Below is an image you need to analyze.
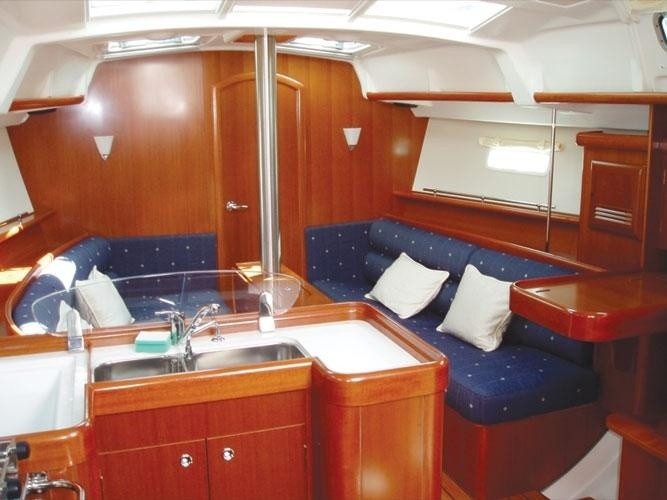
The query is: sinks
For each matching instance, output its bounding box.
[91,326,310,381]
[0,350,89,438]
[292,319,421,374]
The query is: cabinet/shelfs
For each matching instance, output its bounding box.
[510,103,667,500]
[92,390,317,500]
[587,159,645,241]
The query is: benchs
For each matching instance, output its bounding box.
[3,232,234,336]
[302,214,609,500]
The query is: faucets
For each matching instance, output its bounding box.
[65,307,86,353]
[257,290,276,333]
[155,301,221,356]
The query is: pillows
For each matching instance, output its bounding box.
[364,252,450,320]
[55,299,92,332]
[74,265,136,328]
[436,264,515,352]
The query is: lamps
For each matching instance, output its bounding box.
[343,127,362,151]
[94,135,114,161]
